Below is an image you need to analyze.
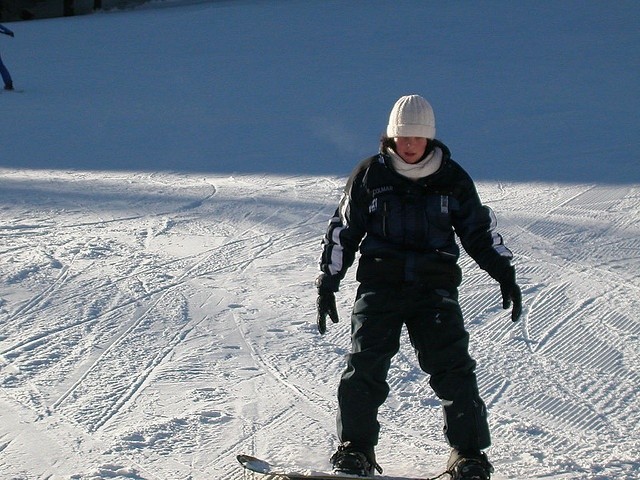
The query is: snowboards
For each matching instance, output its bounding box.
[237,455,437,479]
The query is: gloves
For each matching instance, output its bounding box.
[497,266,522,322]
[315,280,339,334]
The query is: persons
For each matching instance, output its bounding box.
[314,94,522,480]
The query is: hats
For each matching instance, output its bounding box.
[386,94,436,140]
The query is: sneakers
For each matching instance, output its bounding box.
[330,440,383,478]
[446,450,495,480]
